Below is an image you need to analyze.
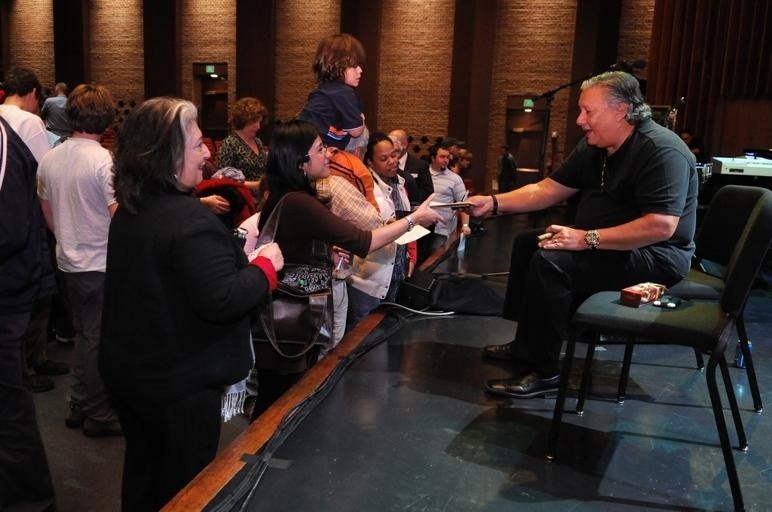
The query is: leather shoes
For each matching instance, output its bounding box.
[483,341,515,361]
[82,416,112,436]
[35,364,49,374]
[30,373,55,393]
[64,409,83,428]
[485,369,561,399]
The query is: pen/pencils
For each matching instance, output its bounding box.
[336,257,344,271]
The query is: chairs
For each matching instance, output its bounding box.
[610,184,772,414]
[547,131,568,177]
[543,191,772,512]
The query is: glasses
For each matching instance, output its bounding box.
[305,145,328,157]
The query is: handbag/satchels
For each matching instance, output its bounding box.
[251,191,334,359]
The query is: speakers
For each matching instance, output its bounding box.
[191,62,228,140]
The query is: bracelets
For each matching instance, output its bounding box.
[492,193,498,216]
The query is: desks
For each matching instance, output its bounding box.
[695,174,772,298]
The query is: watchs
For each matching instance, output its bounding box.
[585,230,602,249]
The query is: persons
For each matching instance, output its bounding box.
[496,144,519,193]
[100,97,473,511]
[1,64,119,511]
[464,71,701,397]
[296,33,366,149]
[677,128,704,159]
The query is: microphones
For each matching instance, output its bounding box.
[673,97,686,109]
[611,59,647,72]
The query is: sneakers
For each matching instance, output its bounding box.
[55,333,79,341]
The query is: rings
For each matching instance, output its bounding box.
[553,238,558,244]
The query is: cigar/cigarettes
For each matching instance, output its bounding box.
[535,232,553,240]
